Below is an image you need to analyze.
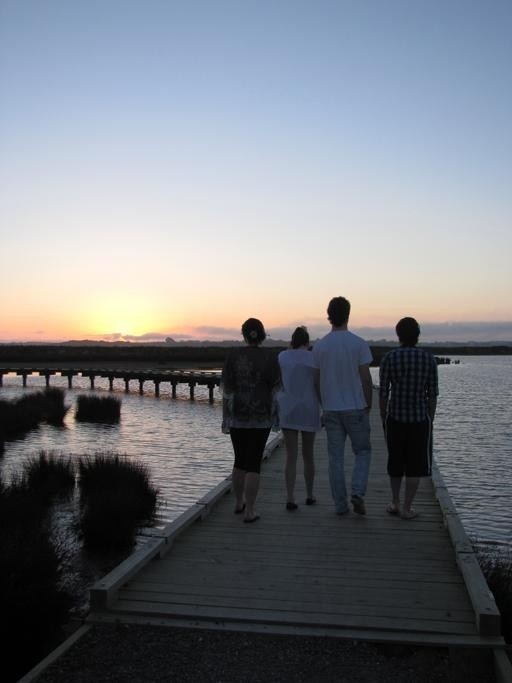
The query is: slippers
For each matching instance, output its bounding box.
[233,502,246,514]
[242,513,261,524]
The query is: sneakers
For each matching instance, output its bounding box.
[400,508,418,520]
[336,507,351,516]
[351,496,366,515]
[386,503,400,514]
[305,495,317,505]
[286,502,298,510]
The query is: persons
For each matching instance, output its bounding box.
[379,315,440,521]
[221,296,372,524]
